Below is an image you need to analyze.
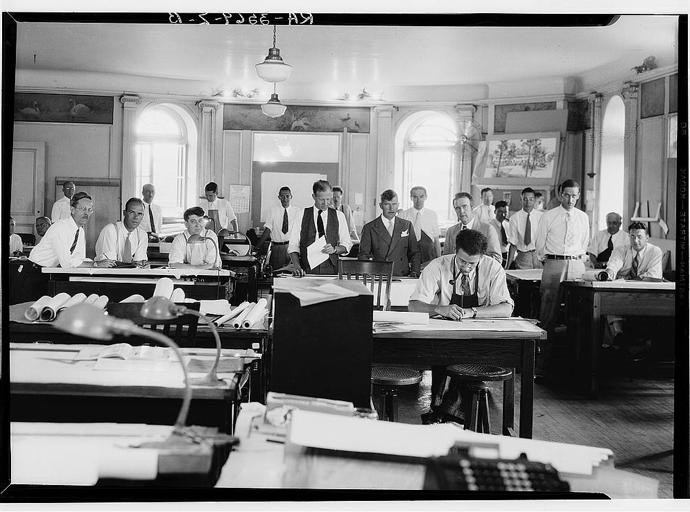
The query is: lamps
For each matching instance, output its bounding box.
[139,295,223,387]
[218,228,253,258]
[203,216,216,234]
[260,80,288,119]
[52,299,209,454]
[186,234,219,270]
[254,26,294,82]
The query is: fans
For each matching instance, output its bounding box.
[442,116,482,192]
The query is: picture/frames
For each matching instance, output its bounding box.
[481,135,560,184]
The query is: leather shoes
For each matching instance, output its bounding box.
[430,395,442,411]
[534,375,545,383]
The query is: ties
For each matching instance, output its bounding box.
[388,221,394,237]
[149,205,156,233]
[70,229,80,255]
[461,275,471,296]
[317,210,325,239]
[190,244,204,265]
[122,233,131,263]
[414,213,422,242]
[629,254,639,280]
[282,208,288,234]
[497,220,508,245]
[597,235,613,263]
[524,213,531,245]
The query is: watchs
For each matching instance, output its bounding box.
[472,306,477,317]
[93,262,97,267]
[333,246,338,253]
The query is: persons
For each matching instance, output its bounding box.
[487,201,518,267]
[397,186,442,257]
[504,188,546,269]
[534,180,590,384]
[36,216,51,239]
[10,216,23,261]
[198,182,239,241]
[606,223,664,281]
[169,208,219,270]
[471,188,497,223]
[408,229,513,409]
[140,184,164,244]
[23,192,93,294]
[589,214,630,270]
[288,180,353,277]
[358,190,421,278]
[328,186,359,243]
[51,183,75,223]
[252,186,304,271]
[443,191,502,265]
[94,197,149,266]
[534,192,546,210]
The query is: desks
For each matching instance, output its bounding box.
[144,253,264,305]
[9,297,270,399]
[580,278,675,385]
[285,315,549,438]
[505,268,554,319]
[17,260,238,304]
[7,344,258,434]
[9,422,239,488]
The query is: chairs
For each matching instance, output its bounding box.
[337,260,394,313]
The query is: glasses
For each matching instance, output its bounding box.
[36,222,48,227]
[64,187,75,190]
[629,234,646,239]
[279,195,292,199]
[75,206,93,213]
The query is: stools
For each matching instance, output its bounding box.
[446,362,512,437]
[372,367,423,427]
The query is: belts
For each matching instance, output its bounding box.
[517,249,536,253]
[547,255,583,260]
[26,259,43,270]
[272,240,289,245]
